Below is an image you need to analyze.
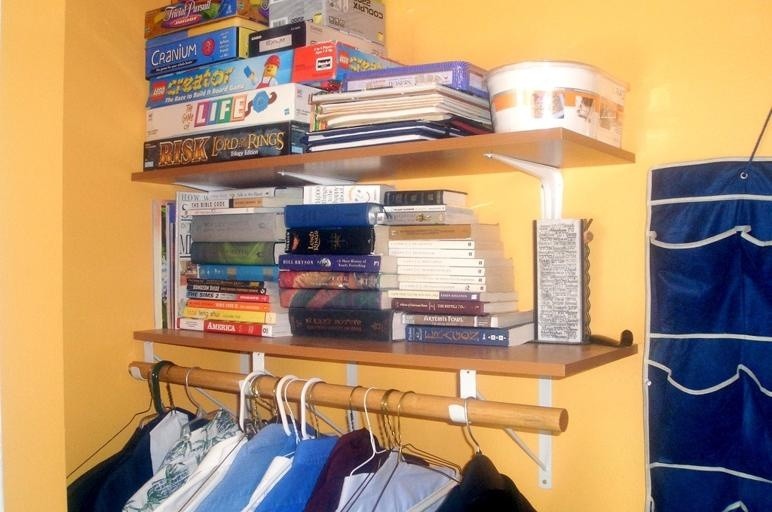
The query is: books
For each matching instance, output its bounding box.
[303,86,493,147]
[150,186,534,349]
[140,1,387,173]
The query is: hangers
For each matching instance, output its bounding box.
[67,359,501,512]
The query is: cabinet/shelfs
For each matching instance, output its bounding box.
[118,125,639,380]
